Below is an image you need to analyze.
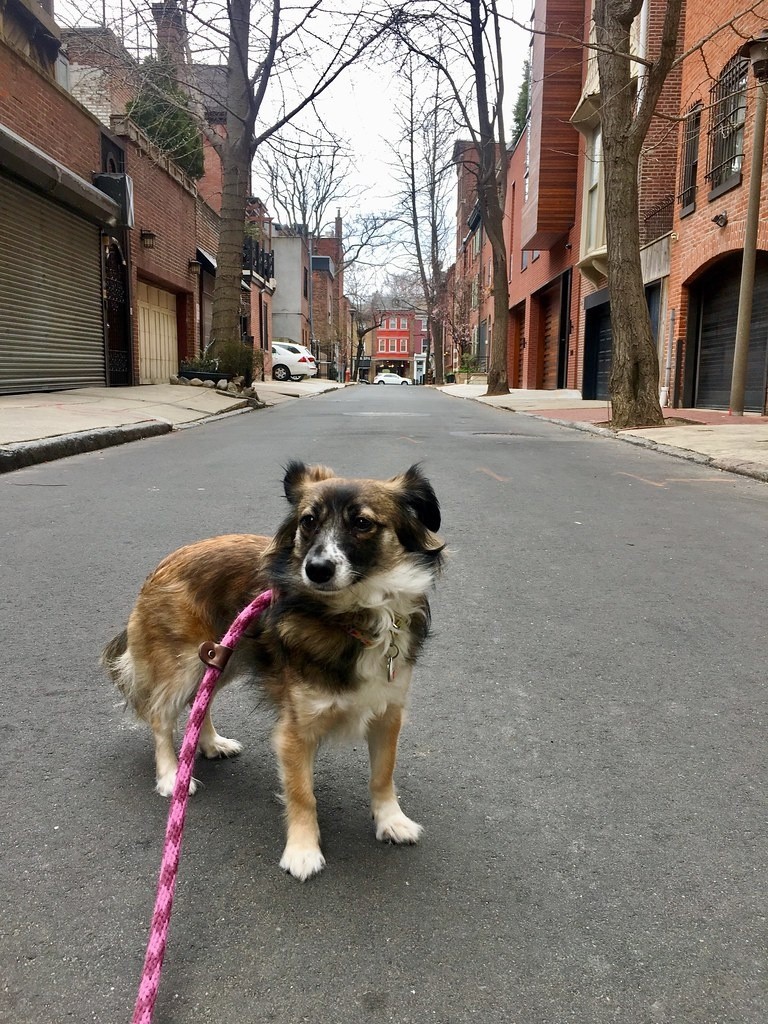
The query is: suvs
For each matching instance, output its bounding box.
[273,341,318,382]
[373,373,413,386]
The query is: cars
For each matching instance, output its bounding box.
[359,379,370,385]
[272,345,309,381]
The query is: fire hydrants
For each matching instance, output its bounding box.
[346,371,351,382]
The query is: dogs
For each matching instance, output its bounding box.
[102,462,458,883]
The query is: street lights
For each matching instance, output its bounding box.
[348,308,356,384]
